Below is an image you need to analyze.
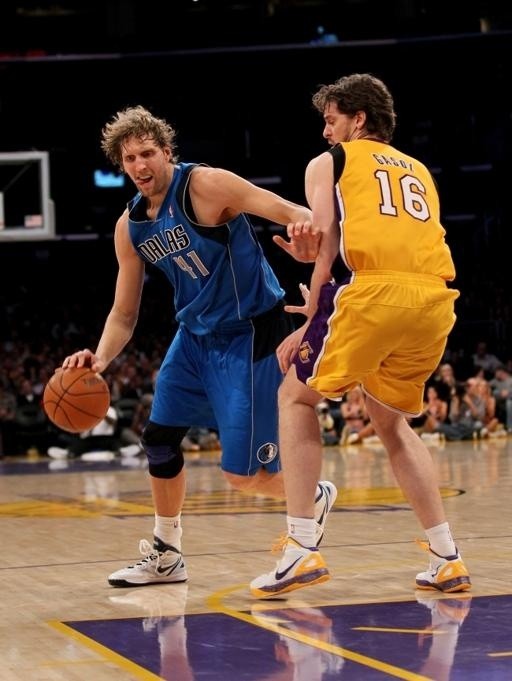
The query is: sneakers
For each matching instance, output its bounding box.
[249,537,330,597]
[416,546,472,592]
[310,481,337,546]
[108,546,187,586]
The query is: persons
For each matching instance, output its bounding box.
[243,74,471,598]
[0,333,512,446]
[62,104,338,585]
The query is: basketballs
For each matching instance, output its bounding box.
[44,368,111,432]
